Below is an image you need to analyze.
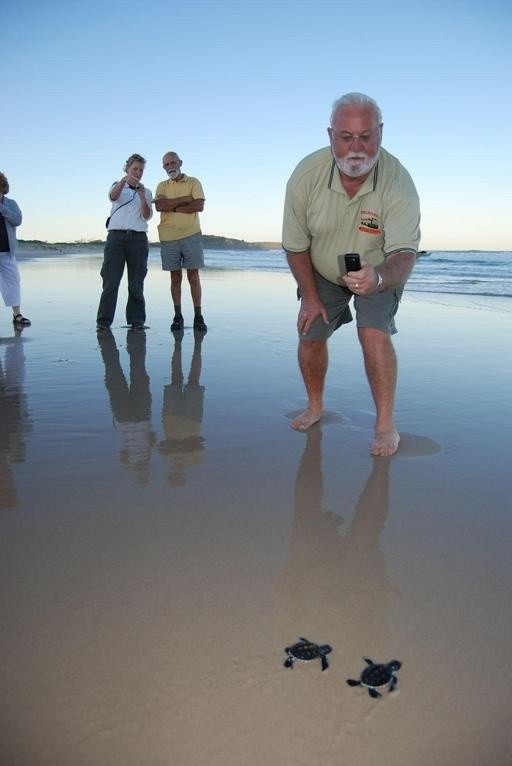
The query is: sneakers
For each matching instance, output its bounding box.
[194,316,207,330]
[171,318,183,329]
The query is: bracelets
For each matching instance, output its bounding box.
[376,273,382,289]
[173,207,176,212]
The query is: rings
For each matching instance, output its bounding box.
[354,284,358,289]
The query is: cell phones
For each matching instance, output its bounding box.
[345,253,361,275]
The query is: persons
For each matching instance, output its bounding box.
[158,331,206,489]
[96,154,153,332]
[150,151,207,332]
[0,172,31,327]
[96,332,157,484]
[0,327,32,463]
[290,421,390,577]
[281,92,421,457]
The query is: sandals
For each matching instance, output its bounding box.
[13,314,31,325]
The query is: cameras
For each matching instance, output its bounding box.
[129,183,140,190]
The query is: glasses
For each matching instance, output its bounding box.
[331,123,383,143]
